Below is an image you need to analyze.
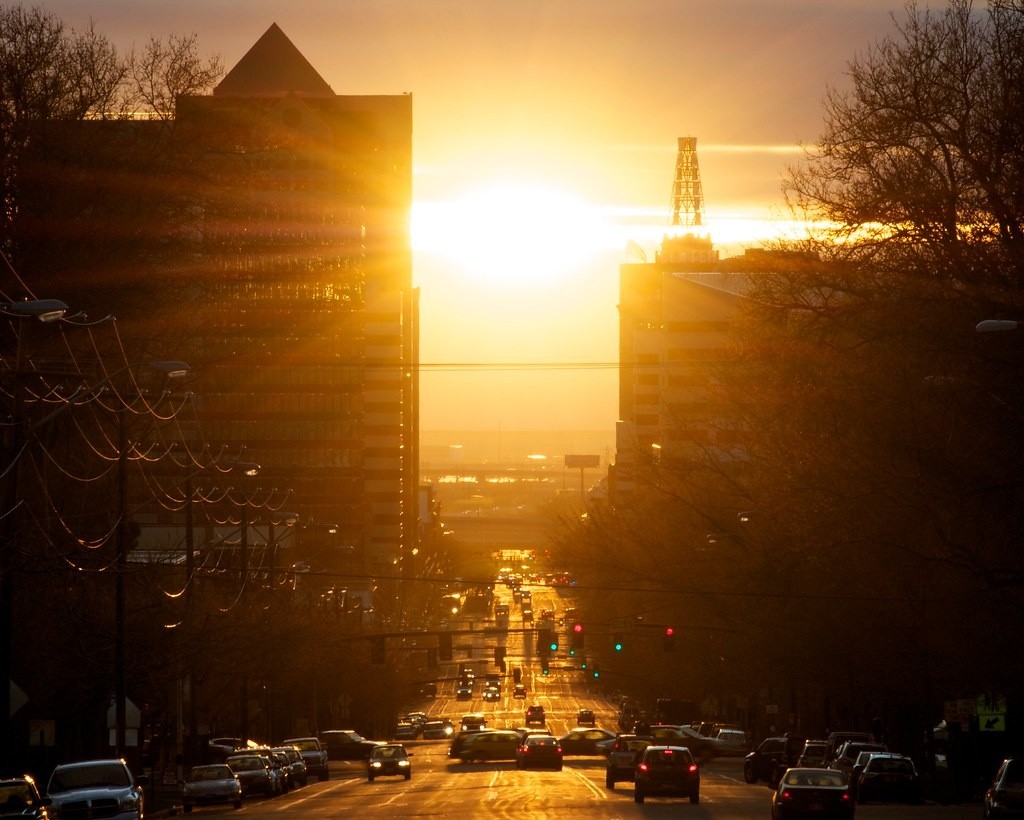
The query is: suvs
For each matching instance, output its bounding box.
[317,729,388,759]
[279,737,330,781]
[524,704,547,726]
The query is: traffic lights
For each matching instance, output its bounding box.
[581,655,587,669]
[663,625,678,652]
[542,663,550,675]
[592,664,599,678]
[572,624,585,650]
[568,647,576,655]
[614,634,624,653]
[548,631,559,652]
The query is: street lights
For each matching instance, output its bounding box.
[262,518,343,736]
[187,456,261,756]
[236,496,297,739]
[111,347,193,755]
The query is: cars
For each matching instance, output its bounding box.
[208,736,254,756]
[494,563,579,618]
[513,685,527,698]
[177,764,243,813]
[0,773,52,820]
[449,726,552,765]
[794,729,923,805]
[43,757,151,820]
[459,715,489,734]
[596,687,750,790]
[741,736,807,786]
[982,754,1024,820]
[394,712,453,739]
[632,745,703,806]
[767,768,855,820]
[417,668,502,701]
[577,708,596,727]
[519,735,564,771]
[223,742,308,799]
[365,744,415,782]
[556,728,616,757]
